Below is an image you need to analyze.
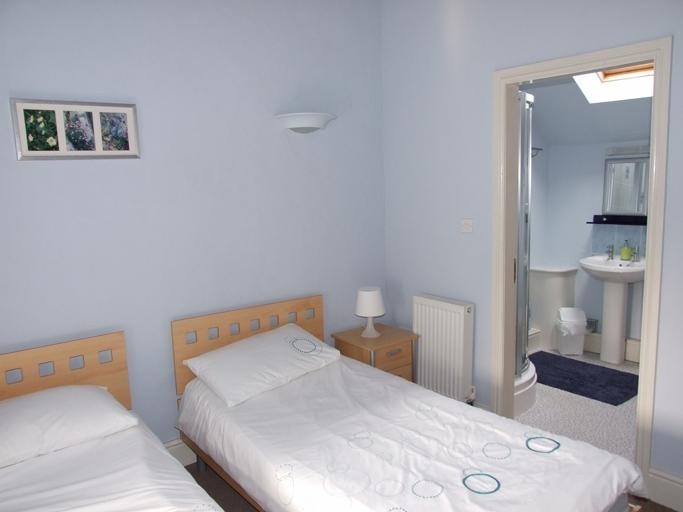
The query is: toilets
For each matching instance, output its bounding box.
[513,360,539,417]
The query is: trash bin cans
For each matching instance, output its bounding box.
[554,307,588,356]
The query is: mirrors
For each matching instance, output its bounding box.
[602,155,649,216]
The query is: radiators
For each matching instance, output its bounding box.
[411,294,478,407]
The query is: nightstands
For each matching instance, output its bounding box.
[330,322,418,383]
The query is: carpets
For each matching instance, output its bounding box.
[524,345,639,406]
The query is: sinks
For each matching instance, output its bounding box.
[578,255,647,284]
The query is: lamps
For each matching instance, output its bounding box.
[276,113,337,132]
[354,285,387,339]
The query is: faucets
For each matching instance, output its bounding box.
[630,246,640,263]
[604,243,614,260]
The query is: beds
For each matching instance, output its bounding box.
[167,294,647,512]
[1,329,228,511]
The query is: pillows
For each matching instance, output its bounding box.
[1,381,142,473]
[177,318,345,407]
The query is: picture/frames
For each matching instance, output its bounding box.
[9,96,141,161]
